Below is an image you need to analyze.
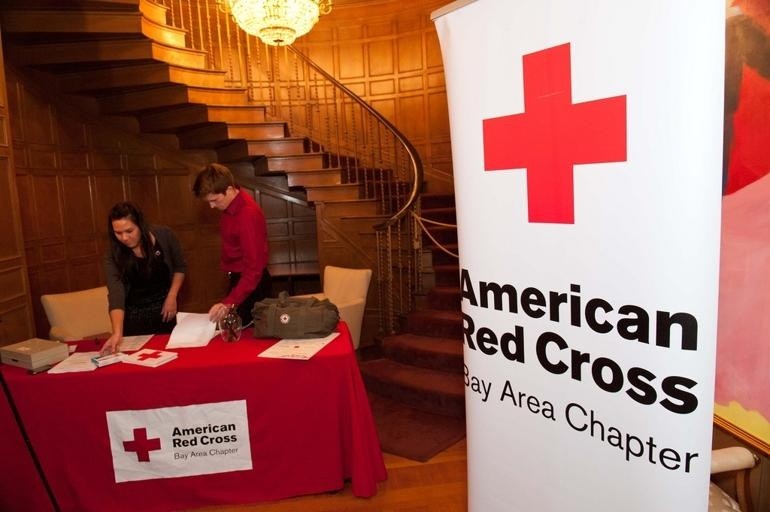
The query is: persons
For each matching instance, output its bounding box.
[190,161,272,328]
[94,200,187,357]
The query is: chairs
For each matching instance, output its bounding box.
[40,286,117,341]
[286,266,373,350]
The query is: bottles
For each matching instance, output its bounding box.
[219,305,242,342]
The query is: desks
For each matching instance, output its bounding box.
[0,319,389,512]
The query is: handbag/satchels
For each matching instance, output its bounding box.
[249,288,340,341]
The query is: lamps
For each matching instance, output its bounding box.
[219,0,334,47]
